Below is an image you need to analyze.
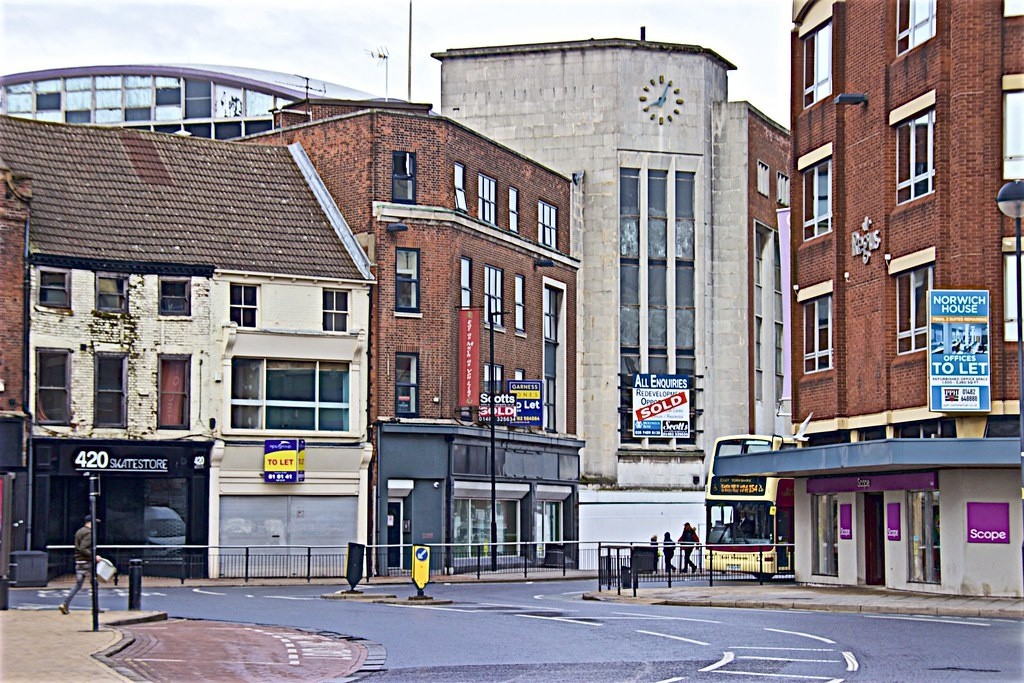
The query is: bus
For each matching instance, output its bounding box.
[704,433,794,581]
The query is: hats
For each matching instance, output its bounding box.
[85,515,100,522]
[652,536,657,540]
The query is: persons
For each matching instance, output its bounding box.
[663,532,676,576]
[678,523,700,574]
[648,536,659,575]
[57,515,105,614]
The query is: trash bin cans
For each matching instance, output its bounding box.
[630,548,657,574]
[13,550,49,587]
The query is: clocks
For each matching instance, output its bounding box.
[637,74,684,125]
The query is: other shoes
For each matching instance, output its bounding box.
[692,566,696,572]
[682,568,687,573]
[92,609,104,613]
[672,567,676,573]
[59,602,69,614]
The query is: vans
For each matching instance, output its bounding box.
[142,506,189,558]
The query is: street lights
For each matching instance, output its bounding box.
[994,181,1024,584]
